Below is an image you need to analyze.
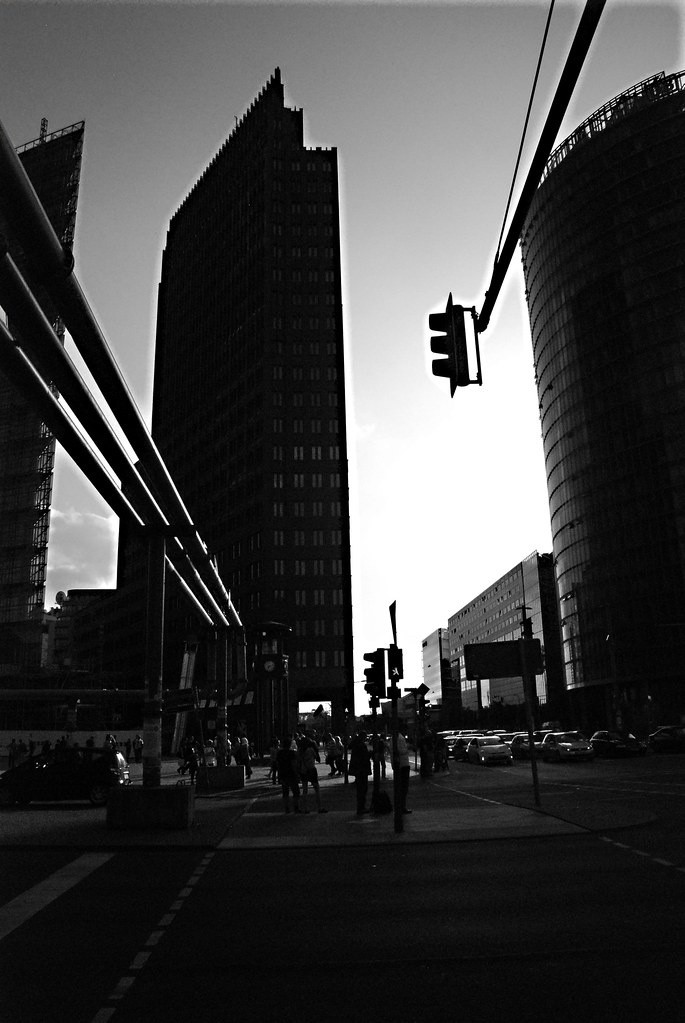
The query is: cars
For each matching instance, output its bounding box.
[589,729,648,757]
[540,730,595,764]
[434,727,556,764]
[648,726,685,753]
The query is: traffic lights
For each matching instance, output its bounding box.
[416,683,431,722]
[388,647,403,681]
[427,292,470,399]
[363,648,386,700]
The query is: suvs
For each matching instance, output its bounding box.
[0,746,134,807]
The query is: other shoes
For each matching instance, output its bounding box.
[402,810,412,815]
[286,809,290,813]
[273,780,277,785]
[318,808,328,813]
[357,808,369,814]
[295,809,303,813]
[305,810,310,813]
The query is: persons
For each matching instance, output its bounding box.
[271,734,329,814]
[176,733,252,786]
[348,730,376,815]
[7,735,67,770]
[385,723,413,814]
[420,721,434,779]
[86,736,95,747]
[103,734,144,763]
[322,732,345,778]
